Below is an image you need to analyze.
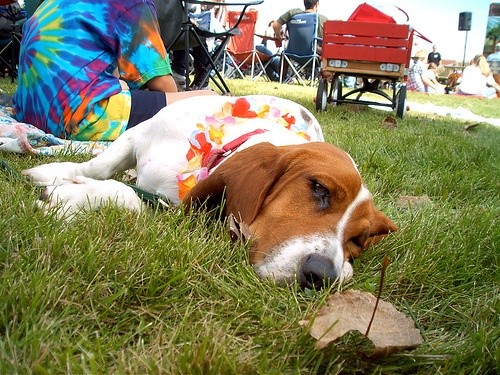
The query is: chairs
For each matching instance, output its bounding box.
[154,0,322,94]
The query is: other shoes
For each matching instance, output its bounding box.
[177,78,186,88]
[192,85,211,91]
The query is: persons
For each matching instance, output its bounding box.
[0,0,27,79]
[15,0,220,140]
[407,45,456,94]
[448,54,500,98]
[265,0,328,76]
[154,0,215,91]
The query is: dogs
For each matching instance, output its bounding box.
[446,71,500,98]
[14,90,401,295]
